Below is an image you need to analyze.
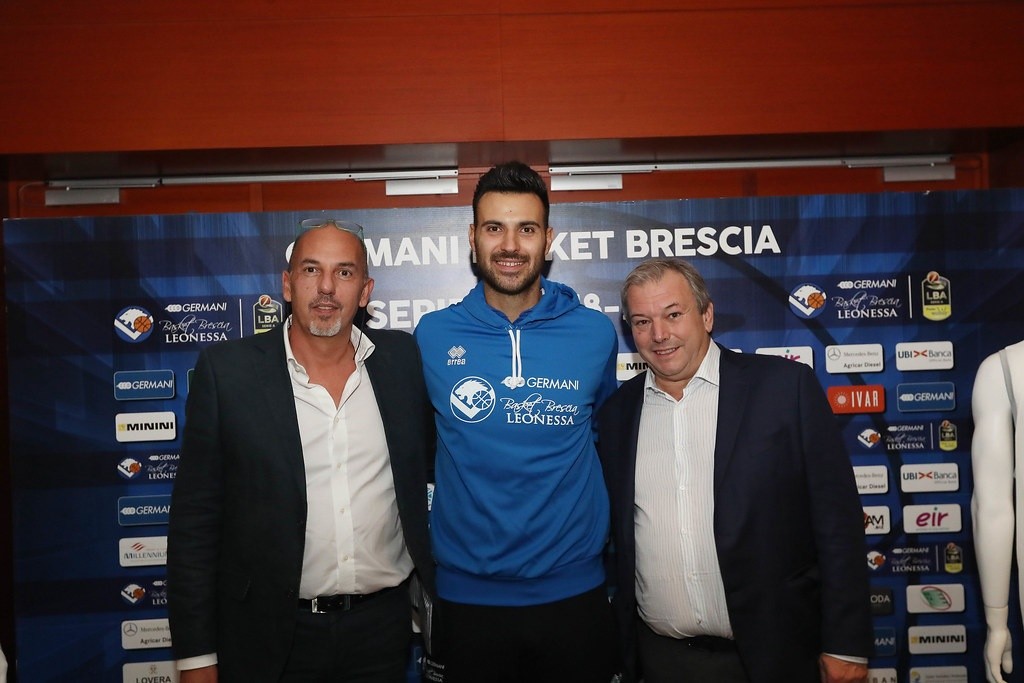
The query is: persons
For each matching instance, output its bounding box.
[960,341,1024,683]
[591,258,870,683]
[413,161,618,683]
[166,224,436,683]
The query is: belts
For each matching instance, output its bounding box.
[297,587,398,616]
[685,634,735,654]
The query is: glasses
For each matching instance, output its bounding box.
[294,217,365,246]
